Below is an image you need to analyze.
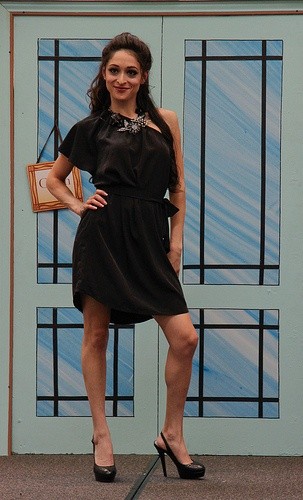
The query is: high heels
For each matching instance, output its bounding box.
[154,431,206,479]
[91,438,116,482]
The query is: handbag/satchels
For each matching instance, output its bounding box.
[27,126,85,212]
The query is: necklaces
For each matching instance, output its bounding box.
[101,106,149,134]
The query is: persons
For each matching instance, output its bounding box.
[45,32,206,482]
